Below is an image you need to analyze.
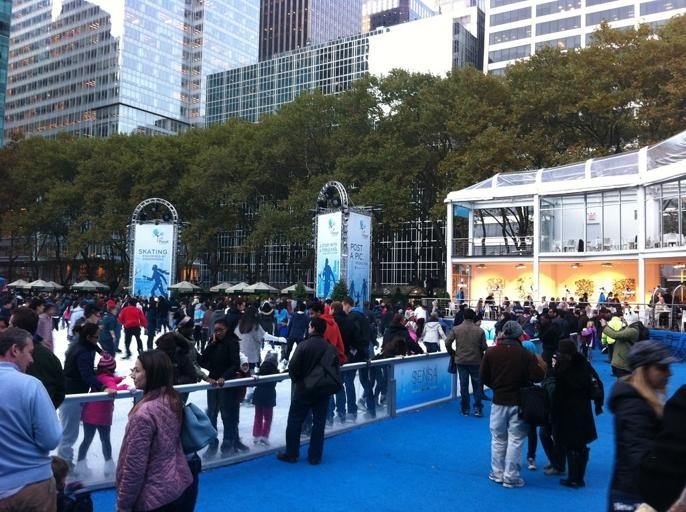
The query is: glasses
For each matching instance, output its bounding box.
[215,328,225,332]
[91,335,98,339]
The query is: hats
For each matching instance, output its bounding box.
[239,352,249,366]
[80,299,88,305]
[281,319,288,322]
[578,310,586,313]
[503,320,522,338]
[98,351,116,371]
[626,339,676,369]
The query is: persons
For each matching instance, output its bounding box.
[1,287,218,511]
[450,287,604,489]
[196,292,449,463]
[602,286,685,512]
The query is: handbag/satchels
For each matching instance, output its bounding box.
[181,399,217,454]
[518,378,550,426]
[303,366,338,399]
[588,376,602,399]
[447,356,458,374]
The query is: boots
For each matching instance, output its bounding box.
[561,448,589,487]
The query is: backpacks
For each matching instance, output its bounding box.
[627,320,649,345]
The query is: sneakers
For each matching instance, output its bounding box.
[543,468,565,475]
[277,453,296,463]
[334,417,345,424]
[326,418,333,427]
[544,463,553,468]
[103,458,117,475]
[488,472,503,483]
[502,478,525,488]
[346,413,356,420]
[259,437,269,446]
[527,458,537,470]
[236,440,250,451]
[254,439,259,445]
[461,410,469,417]
[76,459,91,476]
[474,406,483,417]
[221,438,234,453]
[203,439,219,459]
